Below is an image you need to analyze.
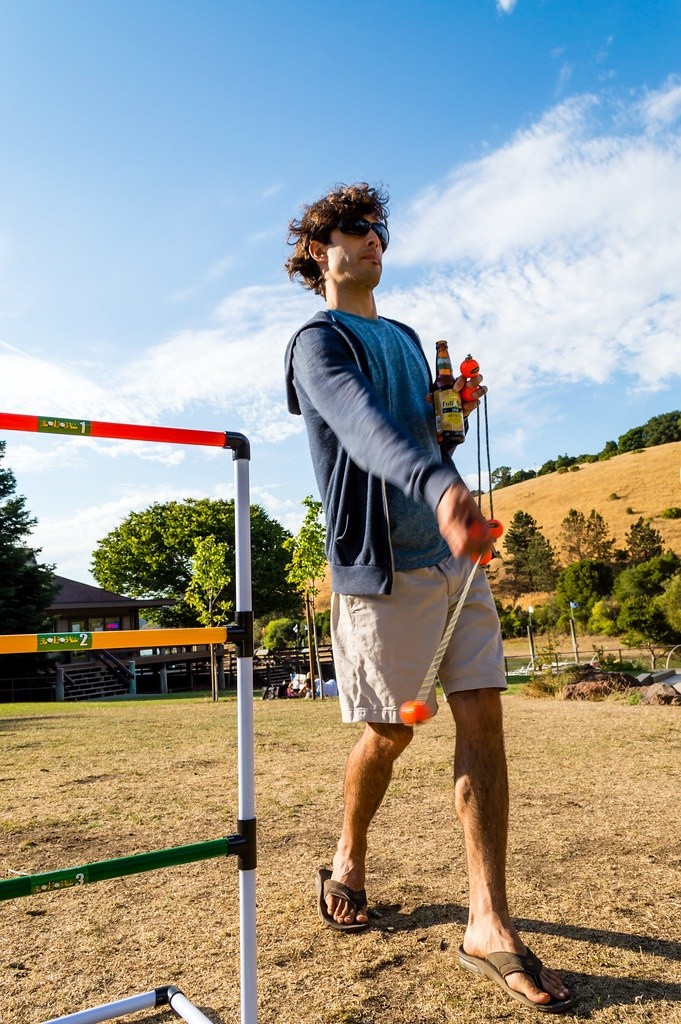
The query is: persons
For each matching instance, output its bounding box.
[278,672,325,700]
[283,183,575,1013]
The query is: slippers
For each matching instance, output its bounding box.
[457,939,575,1013]
[314,868,370,932]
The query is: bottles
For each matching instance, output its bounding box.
[432,340,465,444]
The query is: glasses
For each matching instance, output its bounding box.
[338,216,389,254]
